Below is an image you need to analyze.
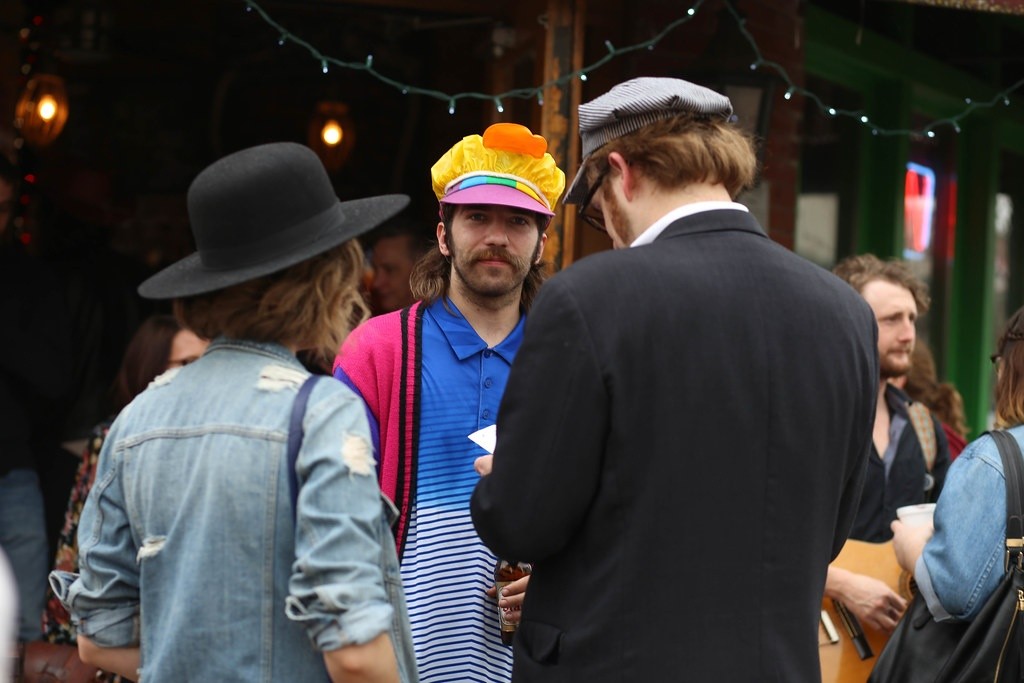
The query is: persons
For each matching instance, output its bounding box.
[469,75,881,683]
[48,141,444,683]
[823,255,969,632]
[0,151,80,683]
[329,120,569,683]
[889,304,1024,624]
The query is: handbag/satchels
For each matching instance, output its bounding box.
[865,428,1024,683]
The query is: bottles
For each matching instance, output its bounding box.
[494,557,532,645]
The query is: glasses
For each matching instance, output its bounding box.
[576,159,632,234]
[990,353,1006,375]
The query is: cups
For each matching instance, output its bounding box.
[896,503,937,530]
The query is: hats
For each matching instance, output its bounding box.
[562,76,734,205]
[430,122,566,232]
[138,143,410,300]
